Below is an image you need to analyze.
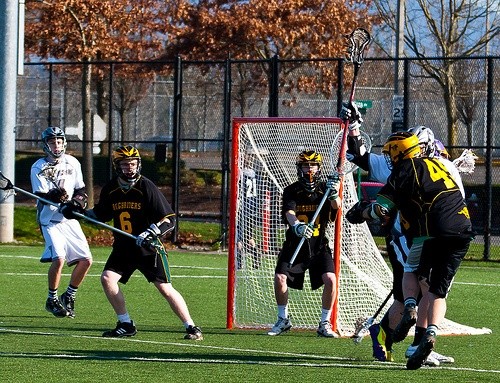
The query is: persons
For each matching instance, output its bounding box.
[234,153,261,270]
[340,99,466,365]
[346,129,471,372]
[30,127,93,319]
[268,149,343,335]
[61,146,205,342]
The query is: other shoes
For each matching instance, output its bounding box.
[393,308,418,344]
[405,334,434,371]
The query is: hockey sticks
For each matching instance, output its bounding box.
[38,167,67,201]
[452,148,480,176]
[353,290,394,343]
[336,27,371,171]
[0,174,162,248]
[290,129,372,263]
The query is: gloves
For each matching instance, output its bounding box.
[136,223,162,251]
[58,202,86,220]
[72,191,88,208]
[295,222,315,239]
[45,188,68,204]
[340,100,363,130]
[345,200,371,226]
[325,180,339,200]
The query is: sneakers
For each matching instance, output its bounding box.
[368,323,395,362]
[405,345,454,366]
[102,319,137,339]
[58,291,76,319]
[45,298,68,318]
[317,320,339,338]
[267,315,292,336]
[183,325,204,340]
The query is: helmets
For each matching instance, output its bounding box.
[408,125,435,158]
[111,145,141,170]
[40,126,66,152]
[381,131,422,163]
[295,149,323,178]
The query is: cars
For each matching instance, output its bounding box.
[137,135,224,153]
